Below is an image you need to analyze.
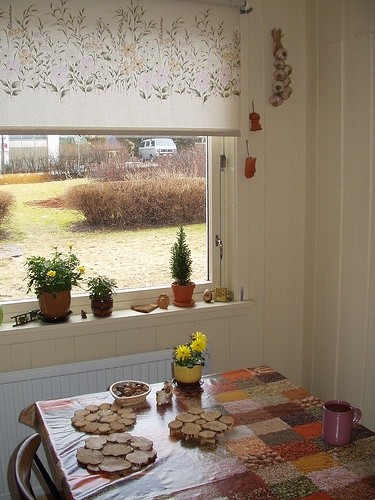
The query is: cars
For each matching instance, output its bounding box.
[66,135,88,149]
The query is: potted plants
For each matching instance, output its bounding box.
[87,275,118,317]
[170,225,196,307]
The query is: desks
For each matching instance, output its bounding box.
[19,365,374,500]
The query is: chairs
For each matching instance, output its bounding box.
[7,432,62,500]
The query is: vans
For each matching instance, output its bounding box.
[137,137,177,163]
[195,136,206,155]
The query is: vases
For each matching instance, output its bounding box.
[174,365,202,383]
[39,290,71,323]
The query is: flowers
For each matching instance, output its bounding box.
[24,242,86,299]
[171,331,211,369]
